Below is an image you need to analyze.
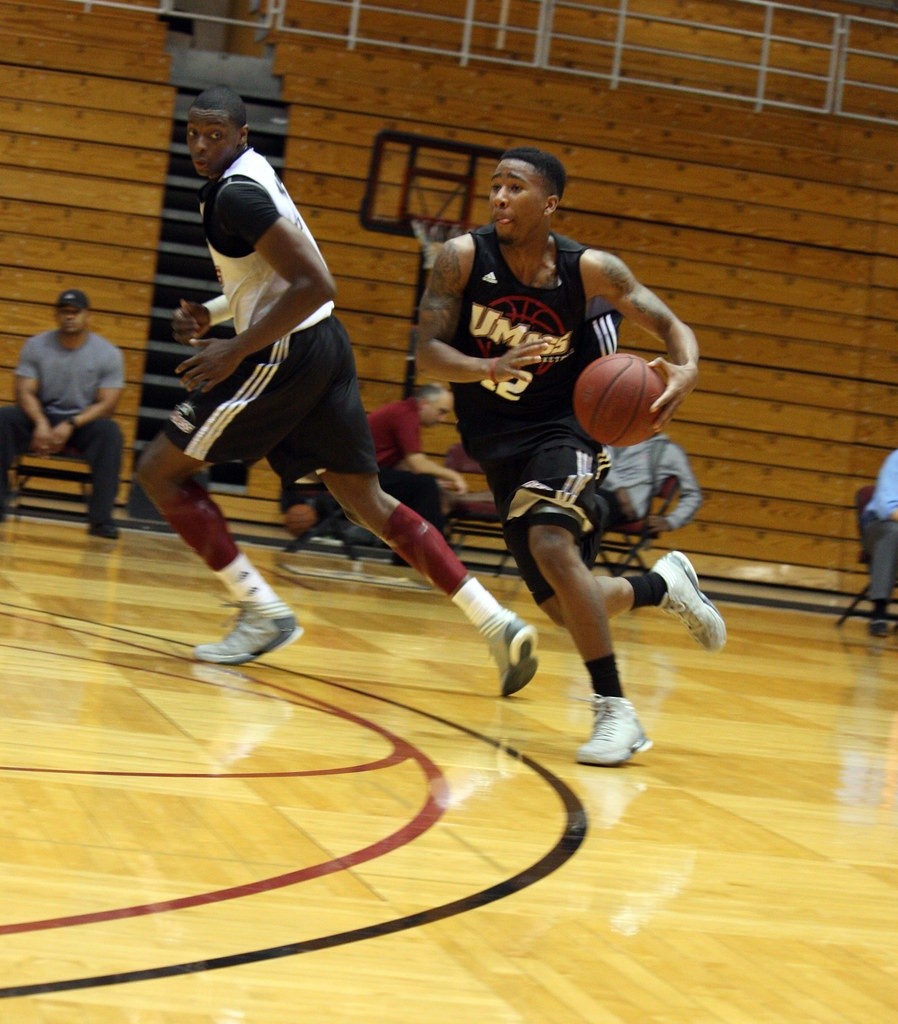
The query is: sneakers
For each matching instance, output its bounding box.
[191,597,306,665]
[573,694,652,766]
[485,613,540,698]
[650,551,728,657]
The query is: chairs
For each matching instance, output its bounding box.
[3,454,94,524]
[600,475,679,576]
[443,444,511,578]
[835,485,898,634]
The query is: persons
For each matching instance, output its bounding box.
[277,379,703,558]
[415,148,740,763]
[866,448,897,637]
[137,88,538,767]
[0,288,125,539]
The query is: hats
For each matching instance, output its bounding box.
[54,289,89,309]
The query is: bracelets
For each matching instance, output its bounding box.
[489,355,499,385]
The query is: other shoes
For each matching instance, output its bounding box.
[88,522,119,538]
[869,619,887,637]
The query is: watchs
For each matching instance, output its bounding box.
[66,415,79,431]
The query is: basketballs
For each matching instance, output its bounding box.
[572,352,666,449]
